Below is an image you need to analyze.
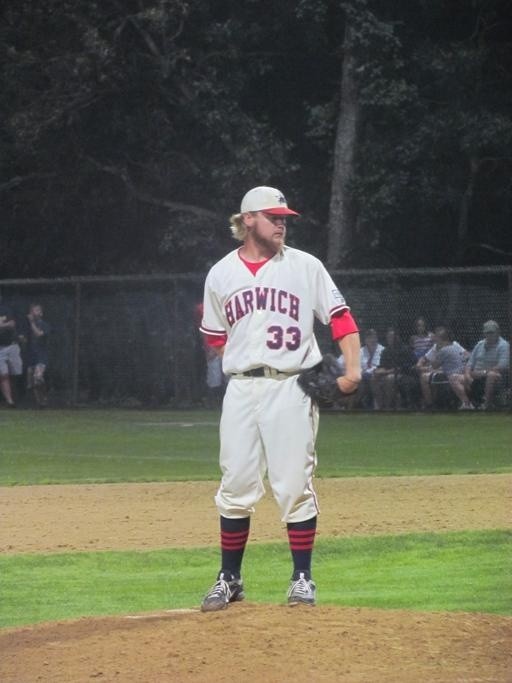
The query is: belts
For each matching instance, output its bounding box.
[231,366,285,379]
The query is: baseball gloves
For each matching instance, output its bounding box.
[298,354,357,404]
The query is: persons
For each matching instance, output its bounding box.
[199,184,367,612]
[18,301,57,409]
[304,314,511,412]
[0,305,24,407]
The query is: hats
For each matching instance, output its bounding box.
[483,320,500,335]
[240,185,302,221]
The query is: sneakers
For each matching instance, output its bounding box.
[460,396,494,410]
[286,569,318,608]
[198,570,246,614]
